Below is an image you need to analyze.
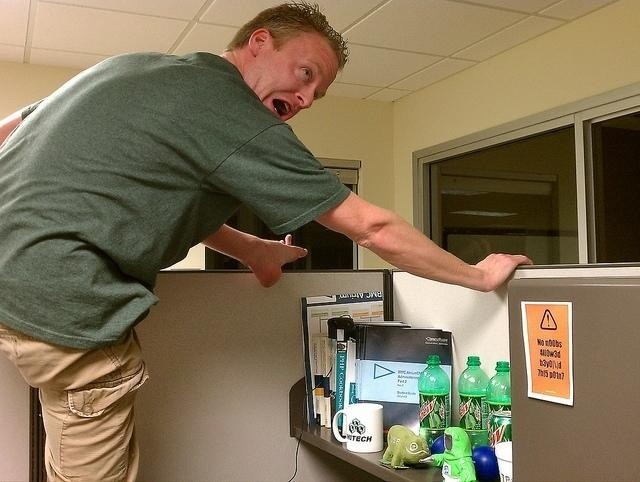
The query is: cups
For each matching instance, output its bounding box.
[332,402,385,453]
[494,442,512,482]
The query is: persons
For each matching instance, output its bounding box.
[0,1,534,482]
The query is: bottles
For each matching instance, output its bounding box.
[418,354,510,451]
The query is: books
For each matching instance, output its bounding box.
[299,290,453,444]
